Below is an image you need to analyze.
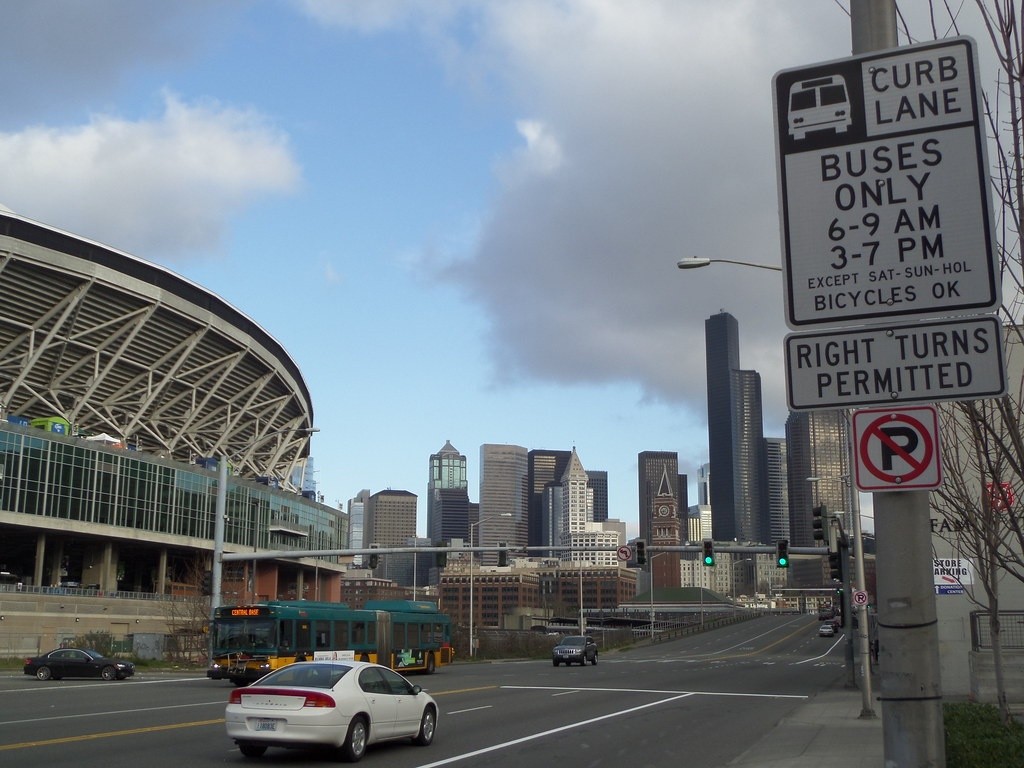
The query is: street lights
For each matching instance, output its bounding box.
[678,255,877,721]
[208,427,323,663]
[650,552,671,638]
[413,535,419,602]
[733,558,752,617]
[470,512,513,657]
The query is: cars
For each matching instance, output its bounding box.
[224,660,439,761]
[24,647,136,681]
[820,625,835,637]
[0,572,23,591]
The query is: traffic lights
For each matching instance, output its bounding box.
[703,541,714,566]
[777,540,790,569]
[814,504,828,541]
[837,585,839,594]
[828,556,841,581]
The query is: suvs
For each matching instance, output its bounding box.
[552,635,599,667]
[818,609,832,620]
[825,619,839,632]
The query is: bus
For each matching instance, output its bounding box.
[203,597,452,686]
[818,605,839,616]
